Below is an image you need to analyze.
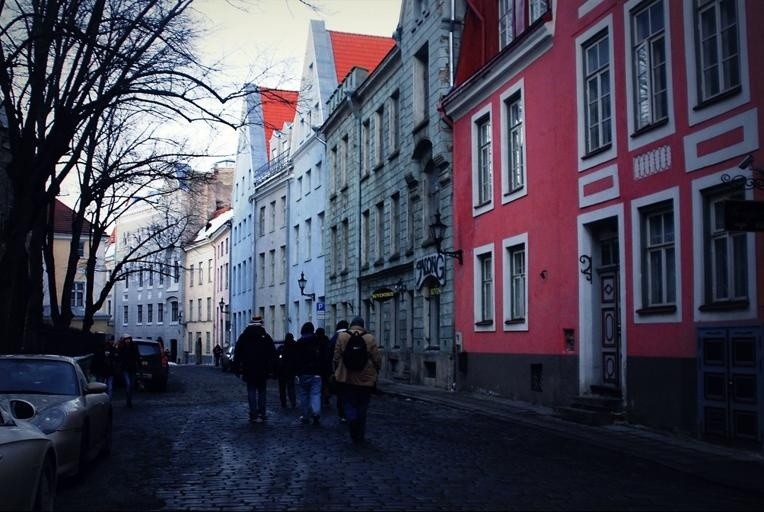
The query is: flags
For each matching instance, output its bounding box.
[105,226,116,244]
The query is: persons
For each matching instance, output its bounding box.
[195,335,203,365]
[234,313,382,446]
[103,332,137,412]
[164,347,171,364]
[212,343,223,366]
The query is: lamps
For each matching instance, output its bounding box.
[430,209,463,265]
[219,298,226,313]
[298,271,315,302]
[178,311,185,325]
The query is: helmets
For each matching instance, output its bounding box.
[120,334,132,345]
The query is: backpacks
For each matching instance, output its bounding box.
[341,331,368,372]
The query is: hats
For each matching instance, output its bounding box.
[248,316,264,327]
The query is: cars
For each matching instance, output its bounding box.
[93,331,171,395]
[220,346,236,372]
[0,353,115,477]
[0,399,57,512]
